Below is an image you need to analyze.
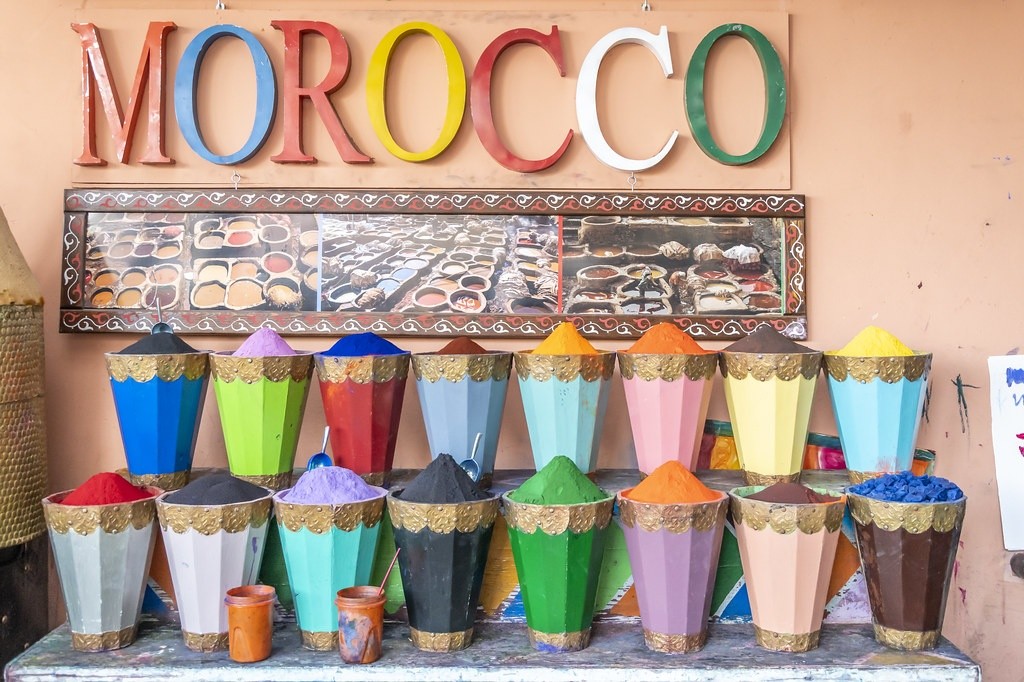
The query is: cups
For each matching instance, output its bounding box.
[273,486,388,651]
[40,485,166,652]
[335,586,386,663]
[514,351,616,482]
[720,350,824,488]
[503,488,616,654]
[729,486,846,654]
[225,584,279,662]
[824,354,932,485]
[209,353,314,493]
[153,486,276,651]
[315,352,411,491]
[386,487,502,652]
[617,487,729,654]
[105,350,214,492]
[411,351,514,490]
[617,349,719,483]
[847,486,968,655]
